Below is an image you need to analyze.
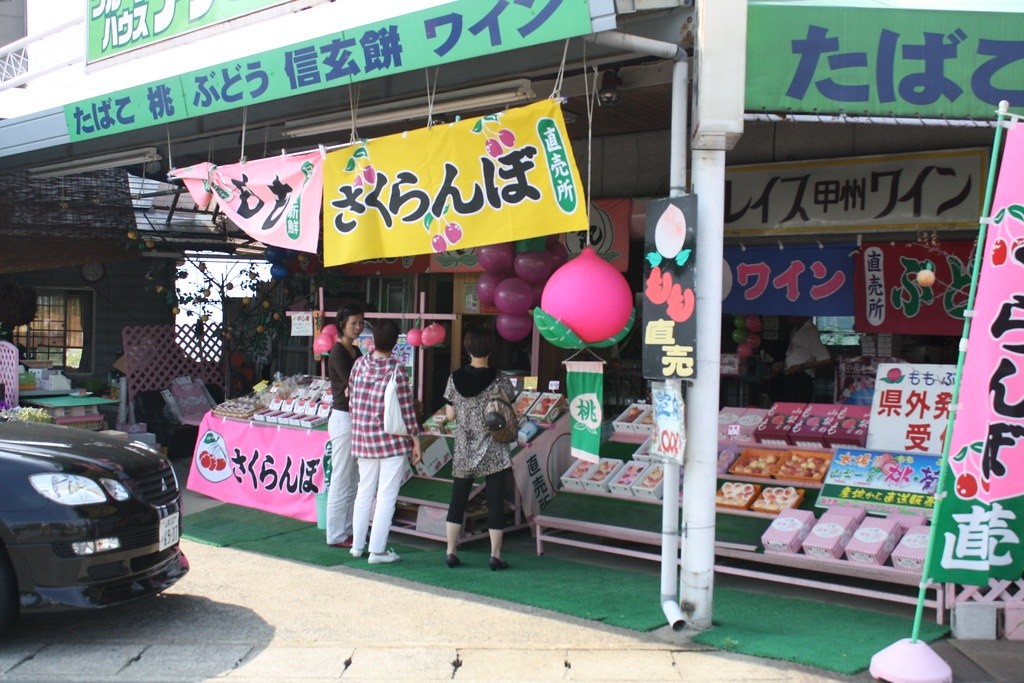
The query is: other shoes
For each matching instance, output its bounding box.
[349,547,363,557]
[330,536,353,547]
[367,547,400,564]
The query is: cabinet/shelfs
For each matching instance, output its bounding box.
[362,395,584,544]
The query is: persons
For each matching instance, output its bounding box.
[347,317,422,565]
[443,327,521,571]
[772,317,831,402]
[327,305,365,547]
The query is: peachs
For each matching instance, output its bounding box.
[533,248,636,350]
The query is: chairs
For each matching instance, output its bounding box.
[162,376,217,426]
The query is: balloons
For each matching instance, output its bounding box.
[732,314,763,356]
[476,237,566,342]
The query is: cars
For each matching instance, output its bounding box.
[0,412,190,638]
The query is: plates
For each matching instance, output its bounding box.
[70,391,94,397]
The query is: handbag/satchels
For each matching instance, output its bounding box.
[383,363,409,436]
[484,372,519,442]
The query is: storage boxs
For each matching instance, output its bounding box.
[560,401,873,514]
[845,516,902,566]
[803,511,857,560]
[762,507,815,554]
[891,523,930,571]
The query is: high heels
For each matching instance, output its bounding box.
[489,557,509,571]
[446,553,459,568]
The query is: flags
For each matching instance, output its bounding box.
[565,361,603,464]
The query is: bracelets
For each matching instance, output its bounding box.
[801,363,805,369]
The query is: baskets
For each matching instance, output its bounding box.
[729,450,834,483]
[210,401,264,419]
[716,484,805,514]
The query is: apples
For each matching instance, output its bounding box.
[567,460,664,490]
[717,455,831,513]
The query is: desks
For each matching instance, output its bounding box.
[187,399,332,523]
